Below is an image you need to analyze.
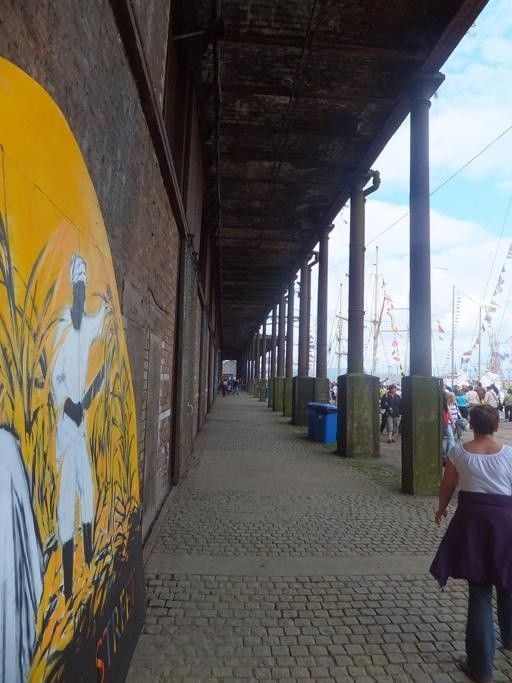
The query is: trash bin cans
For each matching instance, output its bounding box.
[266,388,270,399]
[306,402,337,444]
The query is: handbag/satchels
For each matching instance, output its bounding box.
[505,400,512,407]
[381,406,393,416]
[498,403,503,411]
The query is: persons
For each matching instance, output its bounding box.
[51,252,113,609]
[329,378,512,467]
[429,405,512,683]
[222,378,241,395]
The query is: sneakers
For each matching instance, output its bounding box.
[389,439,397,443]
[459,654,494,683]
[504,419,509,421]
